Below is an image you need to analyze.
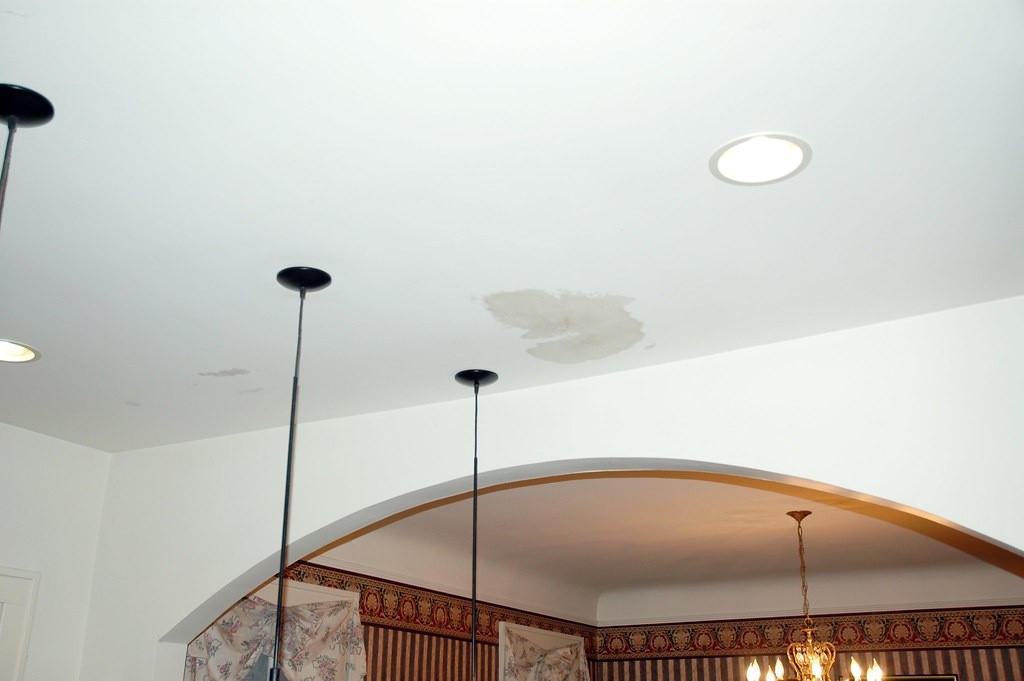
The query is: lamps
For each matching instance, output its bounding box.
[746,507,883,681]
